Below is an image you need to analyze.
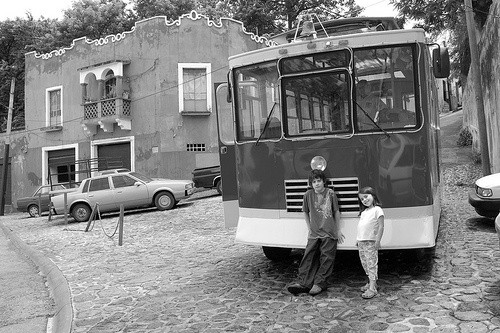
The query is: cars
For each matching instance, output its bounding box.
[48,170,197,222]
[192,165,222,197]
[468,172,500,217]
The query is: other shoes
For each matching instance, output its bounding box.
[362,288,378,299]
[309,284,322,294]
[361,282,378,292]
[287,284,310,293]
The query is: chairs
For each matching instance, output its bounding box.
[260,117,281,141]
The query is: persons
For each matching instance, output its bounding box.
[356,79,387,123]
[355,186,385,298]
[287,169,345,295]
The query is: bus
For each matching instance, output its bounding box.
[17,182,80,218]
[214,0,451,263]
